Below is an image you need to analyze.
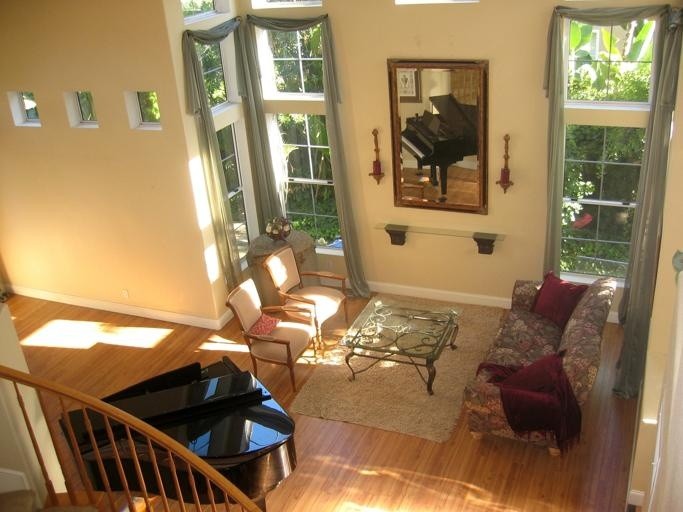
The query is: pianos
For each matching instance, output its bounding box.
[59,357,297,512]
[402,94,479,202]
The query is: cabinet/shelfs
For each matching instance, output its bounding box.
[244,229,318,314]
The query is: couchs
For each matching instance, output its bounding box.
[462,275,618,459]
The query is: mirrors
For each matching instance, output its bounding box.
[385,54,491,218]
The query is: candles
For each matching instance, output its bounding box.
[499,167,509,184]
[372,160,381,175]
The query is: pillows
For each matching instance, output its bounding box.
[500,351,563,394]
[246,312,281,340]
[527,269,591,329]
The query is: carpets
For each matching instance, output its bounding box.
[286,292,507,445]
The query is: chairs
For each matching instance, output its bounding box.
[261,243,350,360]
[401,182,427,202]
[224,276,318,394]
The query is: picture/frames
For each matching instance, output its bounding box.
[395,67,422,104]
